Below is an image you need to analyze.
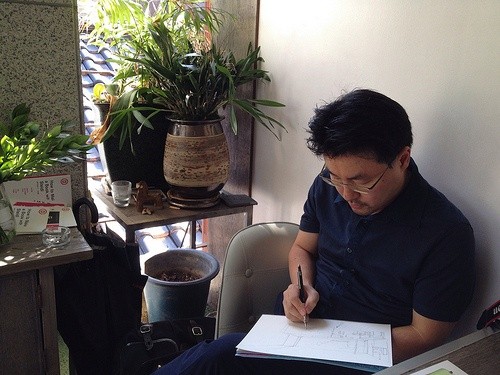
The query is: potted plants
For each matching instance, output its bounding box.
[89,0,288,200]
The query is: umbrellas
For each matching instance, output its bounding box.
[53,198,149,375]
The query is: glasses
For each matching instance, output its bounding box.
[319,151,403,194]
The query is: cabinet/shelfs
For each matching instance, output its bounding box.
[0,228,94,375]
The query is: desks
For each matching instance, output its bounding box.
[90,181,253,250]
[372,326,500,375]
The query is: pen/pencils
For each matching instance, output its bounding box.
[296,264,308,330]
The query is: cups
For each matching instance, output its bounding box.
[110,180,133,208]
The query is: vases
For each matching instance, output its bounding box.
[141,249,220,323]
[0,183,16,253]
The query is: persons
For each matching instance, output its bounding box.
[149,87,475,375]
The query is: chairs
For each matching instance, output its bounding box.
[215,222,301,342]
